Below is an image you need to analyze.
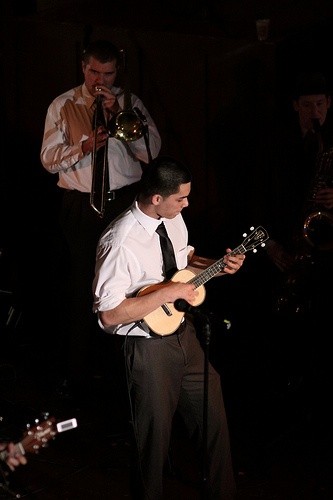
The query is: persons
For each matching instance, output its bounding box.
[92,156,246,500]
[40,39,161,400]
[262,80,333,276]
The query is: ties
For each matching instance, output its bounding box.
[155,221,178,279]
[93,99,110,194]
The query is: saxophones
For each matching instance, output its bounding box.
[278,117,333,314]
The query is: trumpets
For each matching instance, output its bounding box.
[96,87,145,143]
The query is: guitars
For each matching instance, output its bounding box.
[138,225,270,338]
[0,411,78,470]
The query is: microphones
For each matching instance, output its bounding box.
[133,106,147,122]
[174,298,232,330]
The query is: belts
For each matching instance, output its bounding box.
[174,319,187,335]
[81,182,134,201]
